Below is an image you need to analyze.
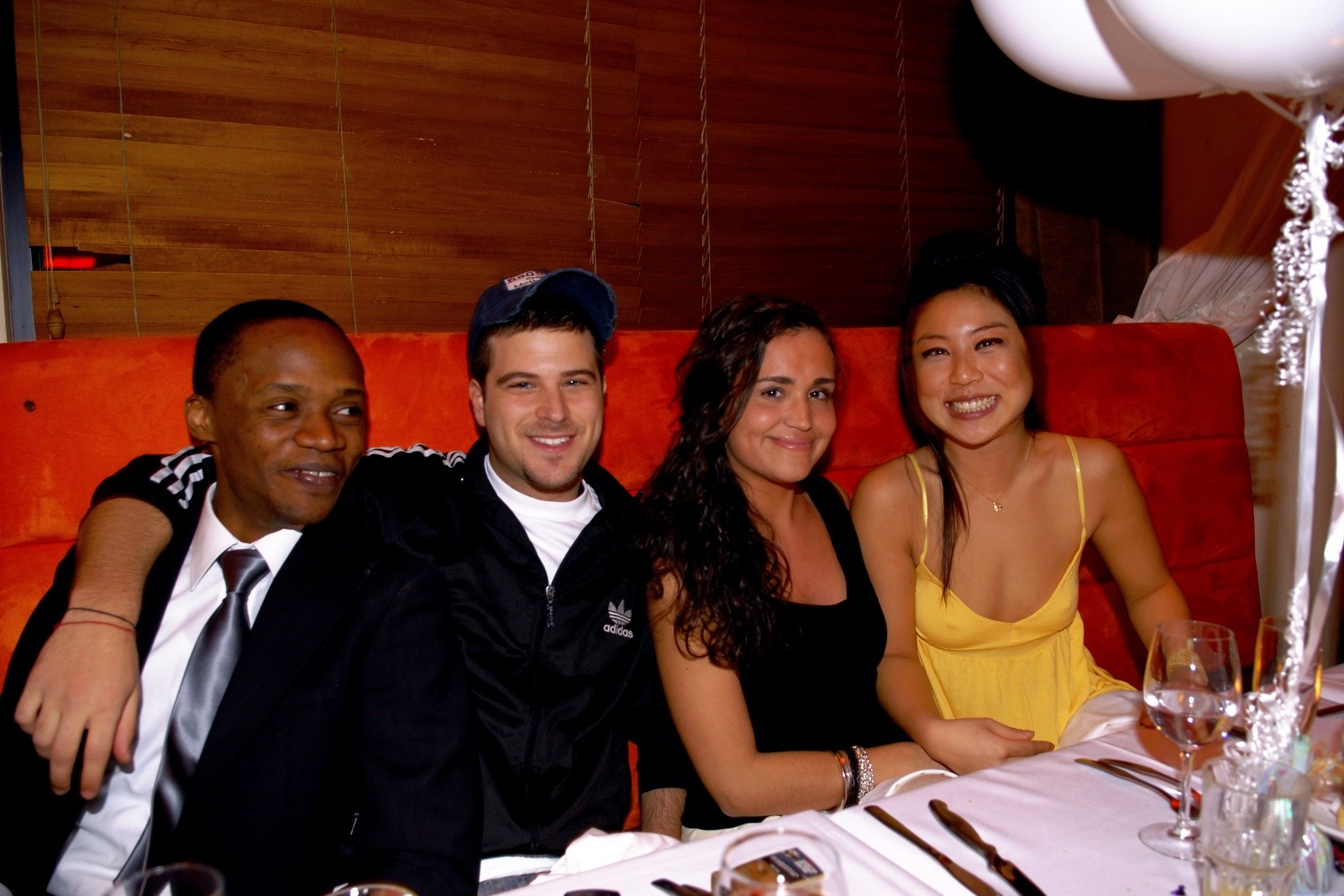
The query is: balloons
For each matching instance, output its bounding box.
[972,0,1344,101]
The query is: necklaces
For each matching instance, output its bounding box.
[950,441,1032,514]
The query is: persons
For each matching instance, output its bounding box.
[850,270,1209,777]
[627,290,960,834]
[14,267,692,860]
[0,300,489,896]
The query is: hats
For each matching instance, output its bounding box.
[470,265,618,342]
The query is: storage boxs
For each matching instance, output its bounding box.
[712,845,825,895]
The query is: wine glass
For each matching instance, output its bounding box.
[1141,619,1246,862]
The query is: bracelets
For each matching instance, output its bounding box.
[54,607,139,630]
[832,746,876,815]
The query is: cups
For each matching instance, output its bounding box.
[324,883,415,896]
[1194,751,1312,896]
[714,830,847,896]
[1250,614,1326,735]
[104,864,226,896]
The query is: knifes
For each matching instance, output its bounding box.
[929,798,1046,896]
[864,804,1002,896]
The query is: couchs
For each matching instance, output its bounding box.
[2,320,1277,829]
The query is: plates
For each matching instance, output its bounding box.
[1309,801,1344,845]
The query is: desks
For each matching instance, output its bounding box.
[485,668,1344,895]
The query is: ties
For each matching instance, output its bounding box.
[113,547,271,896]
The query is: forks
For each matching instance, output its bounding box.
[1075,757,1200,818]
